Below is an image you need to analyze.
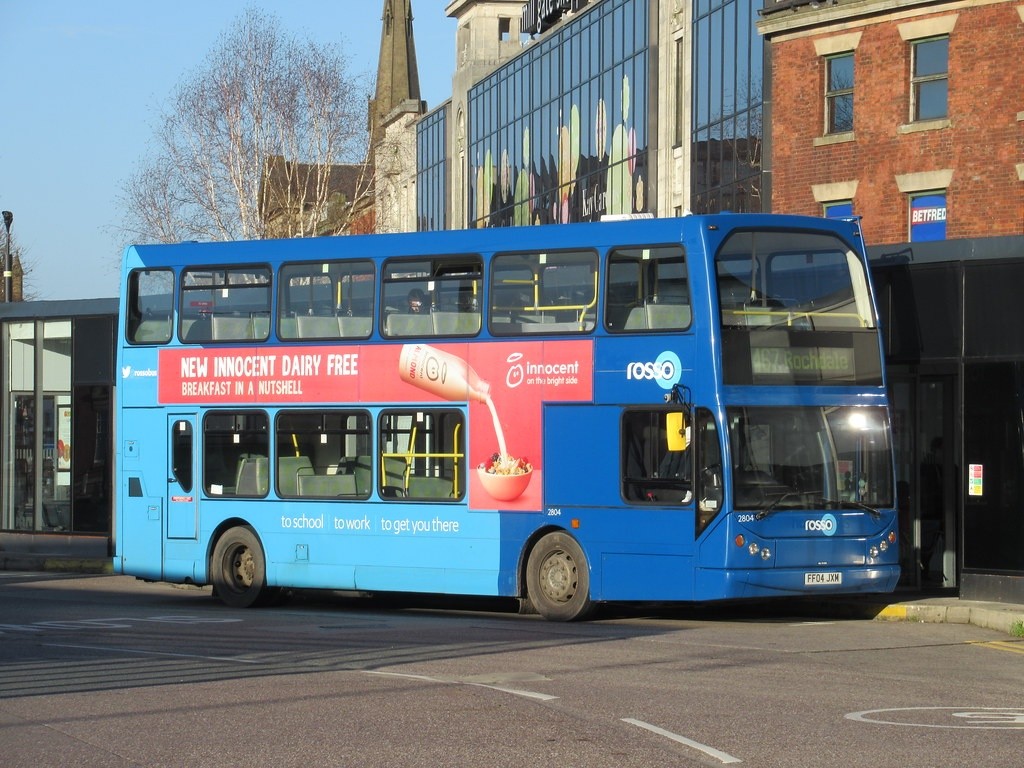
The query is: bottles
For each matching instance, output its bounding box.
[398,344,490,407]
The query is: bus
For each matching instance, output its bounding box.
[108,211,904,624]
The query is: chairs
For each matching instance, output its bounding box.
[175,442,458,499]
[136,305,743,342]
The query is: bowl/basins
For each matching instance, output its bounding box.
[476,462,533,503]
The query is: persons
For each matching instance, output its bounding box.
[921,437,963,580]
[409,288,440,315]
[897,479,913,571]
